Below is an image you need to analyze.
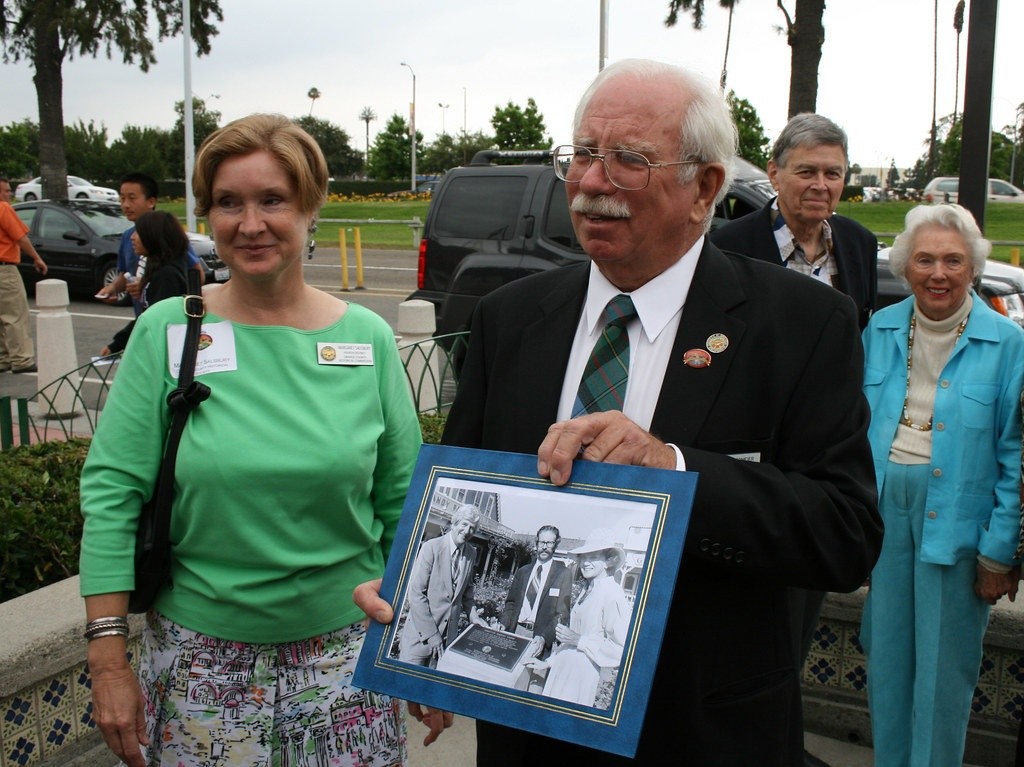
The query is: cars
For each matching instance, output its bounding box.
[14,175,119,202]
[921,177,1024,203]
[9,198,232,306]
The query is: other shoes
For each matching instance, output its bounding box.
[12,364,38,373]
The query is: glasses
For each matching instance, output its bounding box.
[551,144,705,191]
[537,540,557,548]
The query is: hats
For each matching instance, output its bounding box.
[567,528,626,571]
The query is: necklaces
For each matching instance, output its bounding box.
[903,312,968,431]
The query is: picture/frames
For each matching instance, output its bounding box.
[350,442,699,758]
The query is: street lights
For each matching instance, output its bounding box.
[400,62,416,191]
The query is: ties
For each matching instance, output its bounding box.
[526,565,543,611]
[570,294,638,457]
[451,548,461,597]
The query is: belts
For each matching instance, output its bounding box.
[519,623,534,631]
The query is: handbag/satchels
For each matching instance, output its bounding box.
[125,497,173,614]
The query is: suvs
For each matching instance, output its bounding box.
[404,150,1024,353]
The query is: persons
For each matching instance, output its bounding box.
[0,179,47,374]
[80,113,455,767]
[710,113,878,767]
[100,211,189,360]
[861,203,1024,767]
[522,534,633,708]
[399,504,490,670]
[353,61,886,766]
[490,526,572,696]
[95,173,205,319]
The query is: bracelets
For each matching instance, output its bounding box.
[83,617,130,642]
